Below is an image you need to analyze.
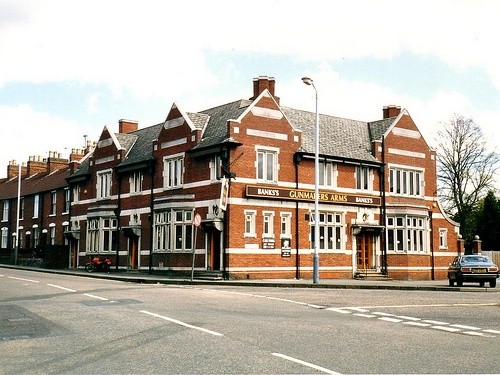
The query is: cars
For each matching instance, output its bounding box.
[448,255,499,289]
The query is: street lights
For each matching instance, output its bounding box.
[427,200,436,280]
[301,77,321,283]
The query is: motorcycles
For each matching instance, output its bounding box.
[85,255,111,274]
